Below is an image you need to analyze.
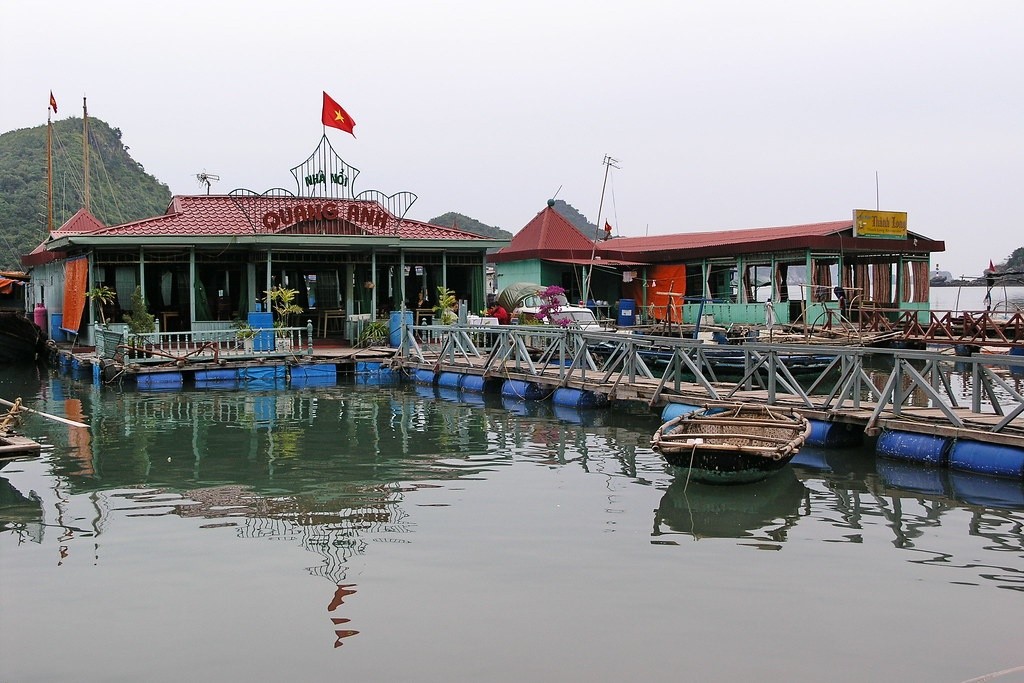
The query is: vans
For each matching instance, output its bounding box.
[510,306,603,357]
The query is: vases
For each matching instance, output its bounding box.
[546,331,559,354]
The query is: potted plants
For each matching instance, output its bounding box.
[126,287,156,358]
[261,287,303,352]
[230,318,255,354]
[360,317,391,344]
[437,285,458,345]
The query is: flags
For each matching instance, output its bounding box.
[322,91,357,140]
[605,221,612,231]
[50,92,57,113]
[988,259,995,274]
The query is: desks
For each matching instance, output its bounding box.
[316,310,346,338]
[569,304,611,325]
[160,311,190,342]
[415,308,436,334]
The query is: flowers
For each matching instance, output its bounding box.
[533,285,572,329]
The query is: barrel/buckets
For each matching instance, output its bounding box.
[195,364,337,381]
[876,429,951,465]
[136,373,183,390]
[661,402,726,428]
[389,311,414,348]
[948,439,1024,477]
[500,379,535,397]
[800,419,836,447]
[248,312,274,350]
[552,387,593,409]
[437,372,464,387]
[413,369,437,382]
[461,374,487,391]
[51,313,67,342]
[617,299,635,327]
[1008,344,1024,374]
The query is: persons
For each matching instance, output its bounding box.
[442,301,459,325]
[487,302,508,326]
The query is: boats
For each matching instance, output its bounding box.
[649,401,812,486]
[0,414,43,457]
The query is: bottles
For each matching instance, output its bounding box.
[355,362,396,373]
[58,354,89,370]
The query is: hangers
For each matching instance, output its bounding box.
[642,279,649,287]
